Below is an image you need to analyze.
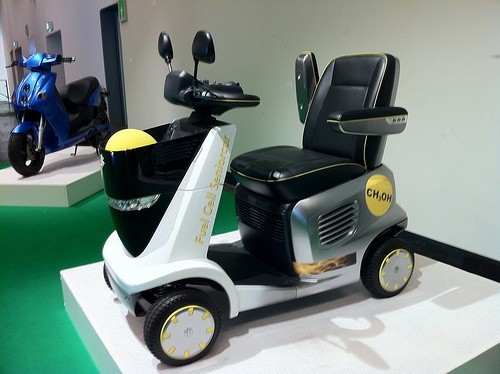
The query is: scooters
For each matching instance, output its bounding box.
[5,52,114,176]
[98,30,416,365]
